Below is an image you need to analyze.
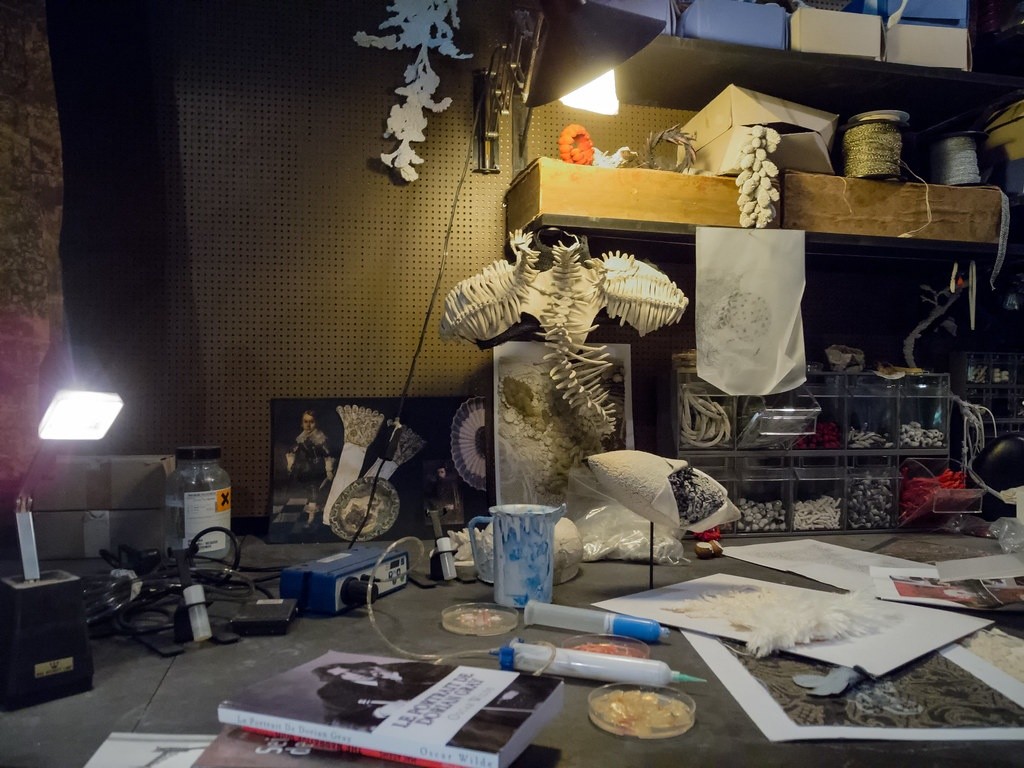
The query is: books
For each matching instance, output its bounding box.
[217,649,565,768]
[84,733,390,768]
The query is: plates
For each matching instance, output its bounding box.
[554,634,650,658]
[587,683,696,740]
[440,601,519,636]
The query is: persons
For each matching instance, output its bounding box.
[425,466,456,517]
[287,412,333,527]
[310,659,457,733]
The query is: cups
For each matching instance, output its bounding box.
[469,503,567,607]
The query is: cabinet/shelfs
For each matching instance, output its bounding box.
[674,353,985,539]
[505,34,1023,270]
[950,350,1024,477]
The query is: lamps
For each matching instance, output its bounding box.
[471,1,668,175]
[0,340,127,710]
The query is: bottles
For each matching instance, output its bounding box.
[163,444,232,561]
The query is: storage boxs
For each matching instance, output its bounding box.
[587,0,973,71]
[31,453,177,512]
[34,508,181,560]
[504,84,1006,245]
